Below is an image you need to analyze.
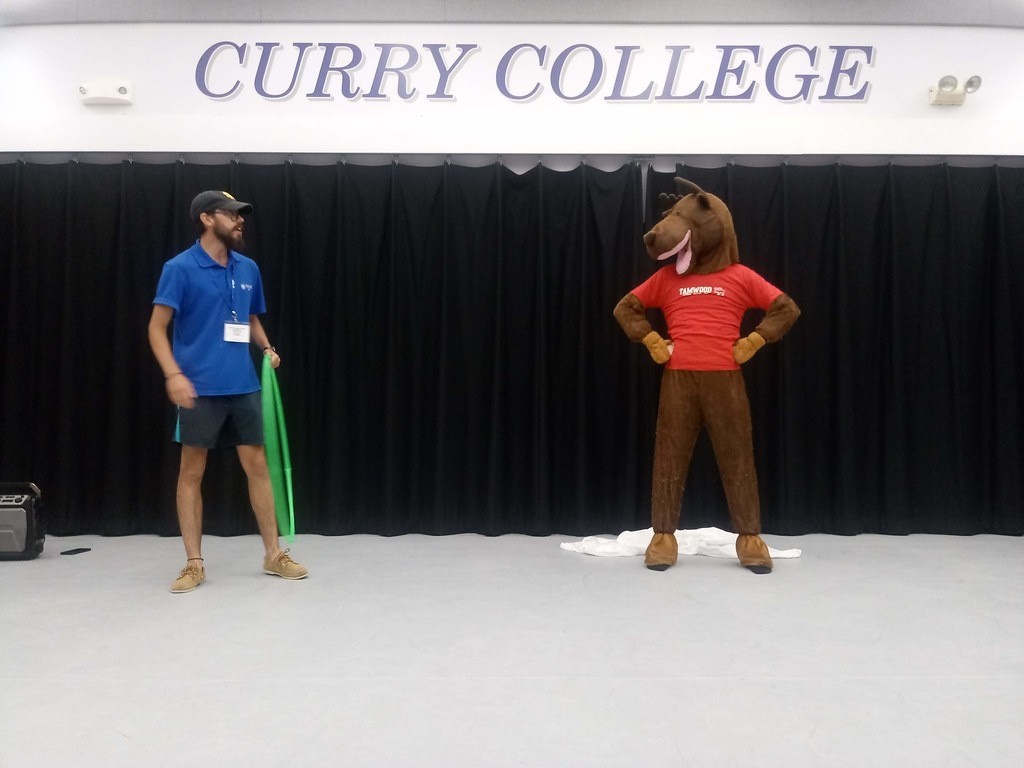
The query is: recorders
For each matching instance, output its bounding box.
[0,481,45,562]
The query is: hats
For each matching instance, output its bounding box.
[189,190,252,223]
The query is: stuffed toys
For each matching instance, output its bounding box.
[612,177,800,573]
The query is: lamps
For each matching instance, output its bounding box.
[928,74,981,104]
[79,83,133,104]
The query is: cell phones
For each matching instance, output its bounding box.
[60,548,92,555]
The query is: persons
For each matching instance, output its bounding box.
[148,189,307,592]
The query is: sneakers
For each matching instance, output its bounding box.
[170,564,205,594]
[263,548,310,580]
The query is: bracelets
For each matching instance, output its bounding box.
[165,372,183,379]
[263,346,275,351]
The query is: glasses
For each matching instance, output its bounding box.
[205,211,241,222]
